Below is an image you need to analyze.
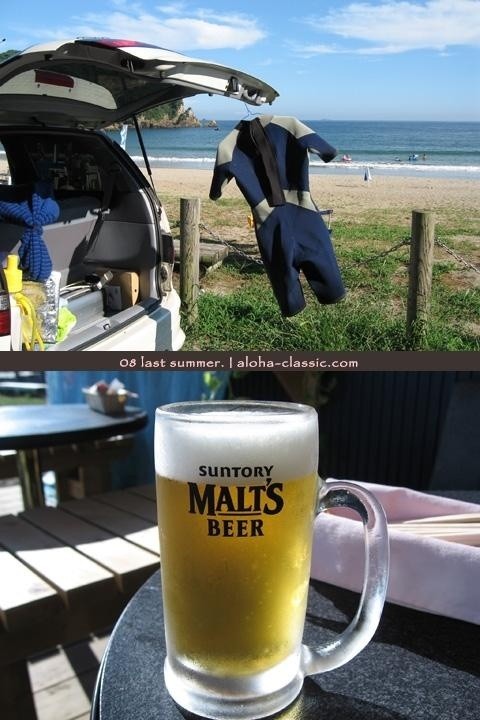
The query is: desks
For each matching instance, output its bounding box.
[0,401,147,515]
[91,552,480,720]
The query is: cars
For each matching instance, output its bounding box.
[0,34,281,353]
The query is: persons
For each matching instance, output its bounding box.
[343,155,350,163]
[396,151,428,162]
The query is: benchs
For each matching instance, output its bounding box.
[0,482,164,644]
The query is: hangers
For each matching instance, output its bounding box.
[218,95,297,146]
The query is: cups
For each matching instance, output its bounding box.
[148,395,392,720]
